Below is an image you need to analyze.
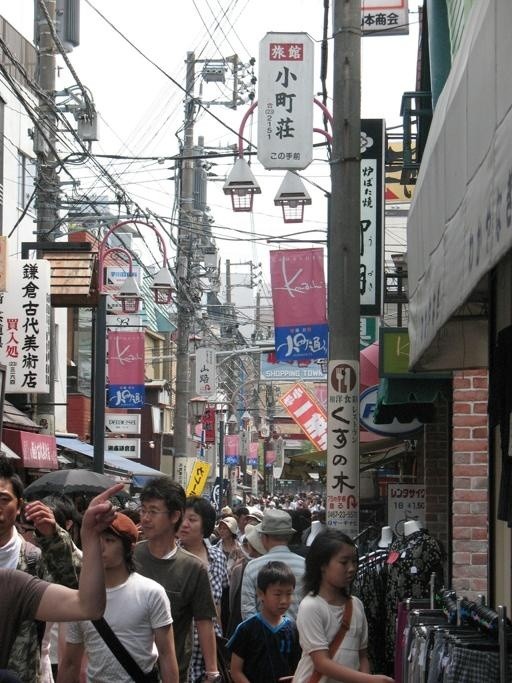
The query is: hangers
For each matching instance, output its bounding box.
[402,588,510,654]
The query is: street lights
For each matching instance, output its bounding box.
[228,414,274,496]
[226,91,359,536]
[93,218,174,468]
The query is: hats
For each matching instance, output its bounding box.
[219,517,239,537]
[108,511,139,543]
[243,509,297,555]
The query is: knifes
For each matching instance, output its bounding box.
[344,368,351,393]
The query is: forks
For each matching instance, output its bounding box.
[336,368,344,393]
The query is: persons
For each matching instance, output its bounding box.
[1,470,392,682]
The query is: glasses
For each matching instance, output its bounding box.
[138,508,171,517]
